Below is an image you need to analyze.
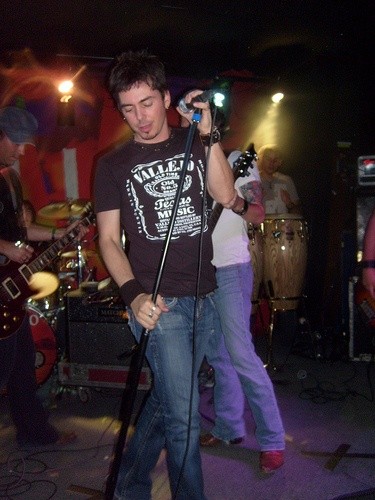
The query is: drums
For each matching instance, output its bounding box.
[24,296,61,391]
[59,268,86,291]
[264,213,311,313]
[247,222,264,317]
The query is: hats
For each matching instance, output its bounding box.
[0,106,39,148]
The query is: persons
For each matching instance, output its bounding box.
[360,209,375,299]
[23,199,50,256]
[255,144,300,214]
[0,105,90,449]
[90,50,235,500]
[181,109,286,472]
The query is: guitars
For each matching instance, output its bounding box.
[0,201,97,341]
[209,142,258,237]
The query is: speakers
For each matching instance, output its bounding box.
[67,290,149,368]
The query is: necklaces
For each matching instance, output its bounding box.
[134,129,174,151]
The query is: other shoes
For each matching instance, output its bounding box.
[16,429,80,449]
[199,433,243,447]
[258,451,285,475]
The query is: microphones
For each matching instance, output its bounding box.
[177,88,216,113]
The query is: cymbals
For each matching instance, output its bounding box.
[37,201,85,220]
[57,250,95,257]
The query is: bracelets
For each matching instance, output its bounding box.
[199,126,223,147]
[51,226,56,240]
[119,280,147,306]
[232,198,248,216]
[361,259,375,269]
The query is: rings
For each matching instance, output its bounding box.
[148,312,154,318]
[24,257,28,261]
[152,305,157,312]
